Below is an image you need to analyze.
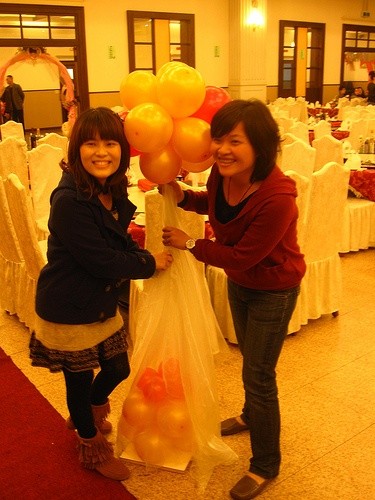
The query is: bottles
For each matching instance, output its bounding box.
[325,113,329,120]
[29,132,37,150]
[369,129,375,154]
[357,135,363,154]
[364,138,370,154]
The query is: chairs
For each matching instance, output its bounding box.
[1,98,373,349]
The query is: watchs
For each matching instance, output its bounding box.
[185,239,196,250]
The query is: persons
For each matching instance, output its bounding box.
[157,98,308,500]
[30,108,174,481]
[333,71,375,105]
[0,74,25,134]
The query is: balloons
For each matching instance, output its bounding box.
[122,358,203,469]
[120,60,234,188]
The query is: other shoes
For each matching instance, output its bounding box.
[230,473,270,500]
[219,417,248,436]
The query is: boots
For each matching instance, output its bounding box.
[77,429,130,480]
[65,398,113,435]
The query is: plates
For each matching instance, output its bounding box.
[134,213,147,225]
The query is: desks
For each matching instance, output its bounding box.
[119,146,215,246]
[321,120,342,130]
[343,167,375,204]
[306,131,349,148]
[308,107,338,120]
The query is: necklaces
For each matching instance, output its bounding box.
[226,176,253,208]
